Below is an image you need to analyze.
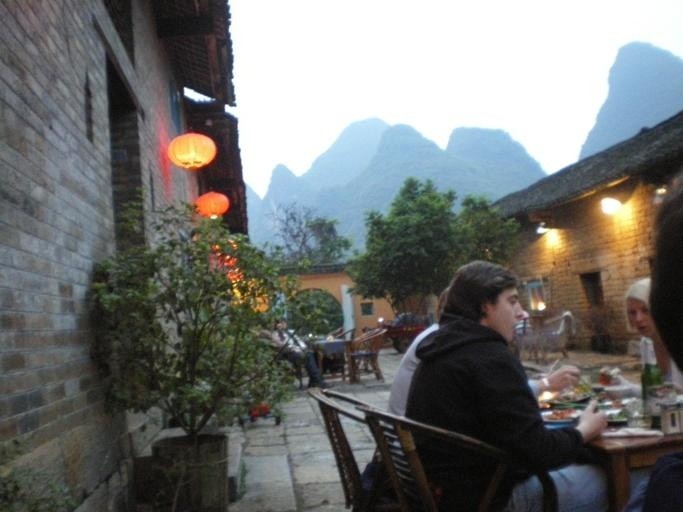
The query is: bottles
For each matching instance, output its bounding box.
[638,337,665,430]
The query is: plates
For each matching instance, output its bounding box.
[538,383,683,427]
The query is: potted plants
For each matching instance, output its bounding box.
[87,185,336,510]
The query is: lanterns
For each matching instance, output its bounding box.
[193,191,230,221]
[167,133,218,172]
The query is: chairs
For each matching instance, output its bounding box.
[308,387,561,512]
[329,325,389,381]
[510,311,575,364]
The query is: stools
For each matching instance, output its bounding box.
[274,349,323,388]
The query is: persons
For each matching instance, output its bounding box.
[272,317,330,388]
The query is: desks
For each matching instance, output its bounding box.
[307,335,346,382]
